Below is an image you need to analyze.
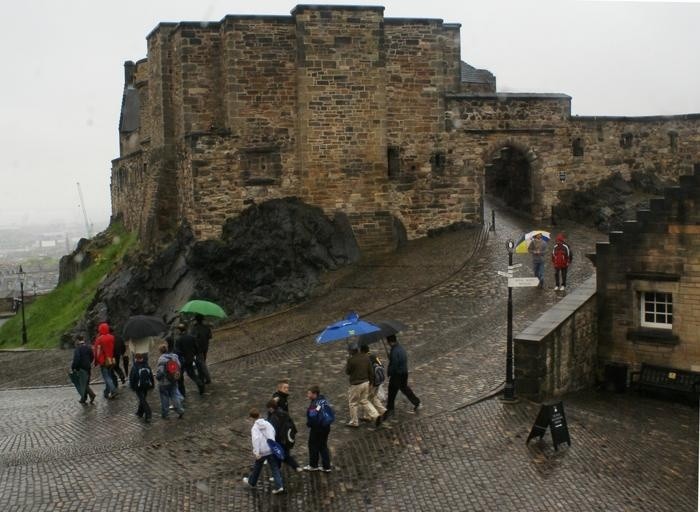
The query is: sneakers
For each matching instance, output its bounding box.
[243,477,256,488]
[554,286,559,291]
[200,383,204,395]
[135,404,184,420]
[272,487,284,494]
[268,477,274,482]
[90,394,95,404]
[79,397,87,403]
[295,464,303,472]
[559,285,564,291]
[345,407,395,427]
[318,466,332,472]
[303,465,319,471]
[103,379,125,399]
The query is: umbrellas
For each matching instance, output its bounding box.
[317,312,381,358]
[357,320,408,360]
[516,230,551,254]
[178,299,230,325]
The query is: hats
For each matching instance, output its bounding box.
[193,315,205,320]
[343,343,358,350]
[175,323,187,330]
[555,233,564,242]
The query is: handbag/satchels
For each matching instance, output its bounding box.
[267,439,286,460]
[105,357,116,370]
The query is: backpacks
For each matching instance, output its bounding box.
[369,354,385,386]
[270,411,297,449]
[157,353,184,384]
[132,361,153,389]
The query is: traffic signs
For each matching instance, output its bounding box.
[506,277,541,288]
[497,271,513,278]
[508,268,521,273]
[508,263,522,269]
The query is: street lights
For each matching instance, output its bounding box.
[15,264,28,345]
[32,280,38,295]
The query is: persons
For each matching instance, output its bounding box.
[71,314,212,421]
[528,233,547,289]
[345,335,420,428]
[551,233,572,291]
[243,380,332,493]
